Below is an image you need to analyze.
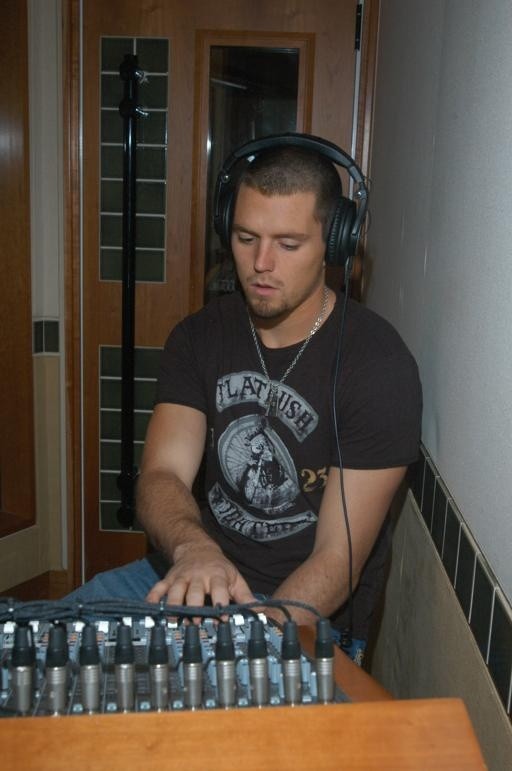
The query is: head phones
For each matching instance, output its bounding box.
[212,132,369,271]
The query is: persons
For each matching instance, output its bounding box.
[40,143,424,667]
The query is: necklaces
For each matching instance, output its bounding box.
[244,284,329,419]
